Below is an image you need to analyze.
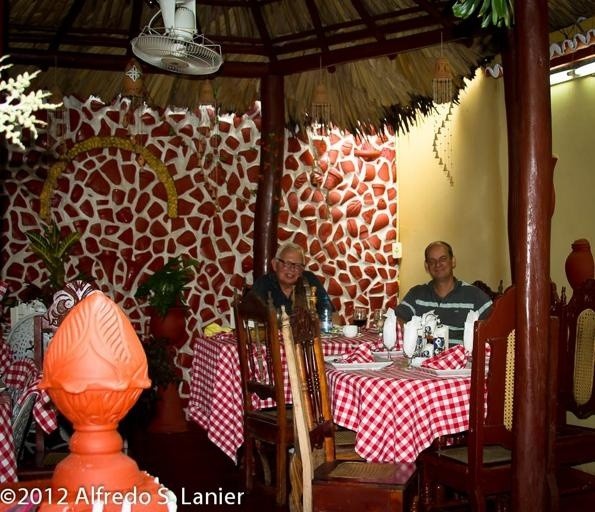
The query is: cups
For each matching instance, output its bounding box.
[343,325,358,339]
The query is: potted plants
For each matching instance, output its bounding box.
[135,256,200,340]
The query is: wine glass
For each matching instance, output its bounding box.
[463,341,472,368]
[404,344,418,371]
[354,308,367,338]
[375,309,384,340]
[384,340,398,362]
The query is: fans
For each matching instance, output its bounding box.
[130,0,224,75]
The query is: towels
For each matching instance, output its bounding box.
[421,344,468,370]
[333,342,375,364]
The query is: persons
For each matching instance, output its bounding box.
[394,240,495,349]
[239,242,335,323]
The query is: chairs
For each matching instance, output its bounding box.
[559,278,595,511]
[280,286,421,512]
[421,284,559,512]
[18,279,94,481]
[233,286,295,507]
[1,358,38,471]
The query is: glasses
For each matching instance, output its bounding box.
[277,258,305,272]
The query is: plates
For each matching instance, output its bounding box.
[373,351,406,362]
[411,362,472,376]
[331,358,395,372]
[319,334,339,339]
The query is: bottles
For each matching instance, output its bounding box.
[321,293,333,333]
